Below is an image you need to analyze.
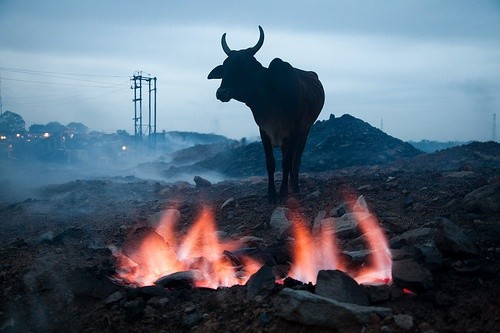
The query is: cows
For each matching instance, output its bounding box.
[207,25,326,205]
